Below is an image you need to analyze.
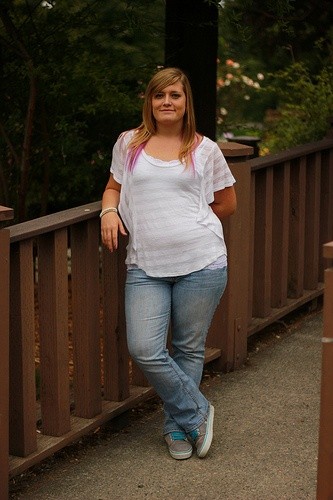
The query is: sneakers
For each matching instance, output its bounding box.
[164,430,193,460]
[187,404,214,458]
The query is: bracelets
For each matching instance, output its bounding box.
[99,207,118,218]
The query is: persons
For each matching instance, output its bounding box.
[100,66,237,460]
